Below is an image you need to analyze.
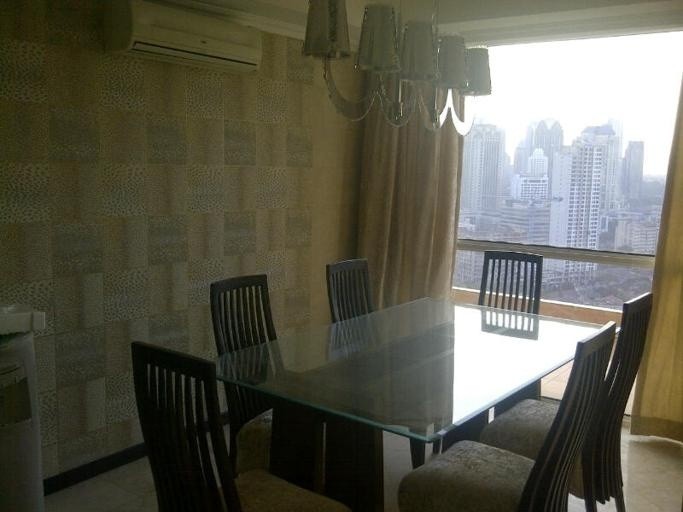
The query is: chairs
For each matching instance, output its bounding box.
[130,339,351,512]
[209,274,285,474]
[477,250,544,401]
[480,291,655,511]
[397,320,616,512]
[326,258,379,347]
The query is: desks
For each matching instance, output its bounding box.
[211,296,623,511]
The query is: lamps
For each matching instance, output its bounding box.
[302,0,492,137]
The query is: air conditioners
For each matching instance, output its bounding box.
[103,0,262,77]
